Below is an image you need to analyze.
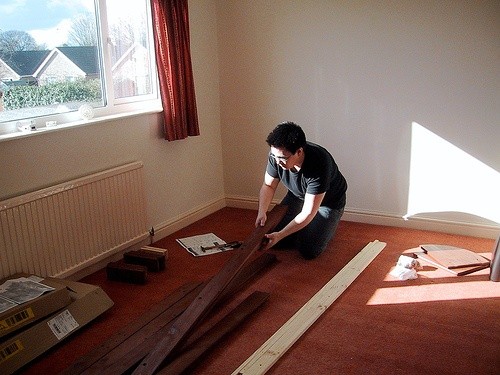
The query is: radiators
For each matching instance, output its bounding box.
[0,160,151,279]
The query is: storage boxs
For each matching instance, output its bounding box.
[0,271,116,375]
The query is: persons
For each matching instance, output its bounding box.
[255,121,348,260]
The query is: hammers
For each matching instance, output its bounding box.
[200,243,226,252]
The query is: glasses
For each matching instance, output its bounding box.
[268,148,293,162]
